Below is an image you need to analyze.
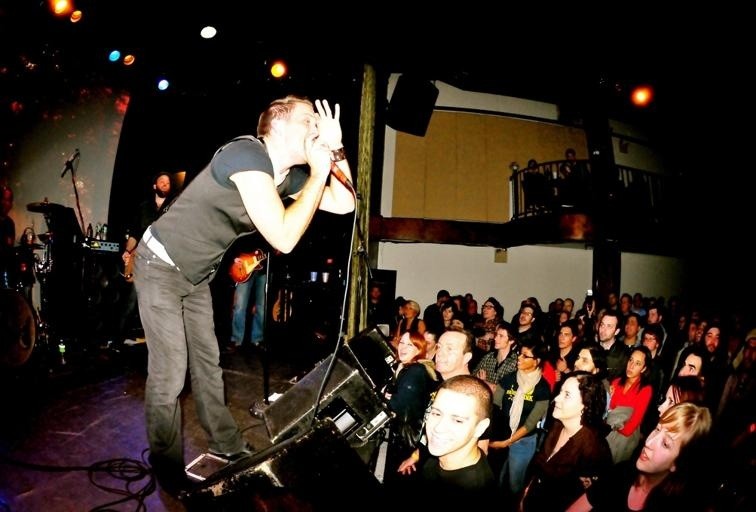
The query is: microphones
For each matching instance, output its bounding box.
[61,153,79,178]
[331,161,350,188]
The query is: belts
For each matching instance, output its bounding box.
[142,221,181,272]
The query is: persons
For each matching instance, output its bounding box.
[367,285,756,512]
[3,189,37,292]
[123,170,174,345]
[224,233,278,352]
[135,95,354,498]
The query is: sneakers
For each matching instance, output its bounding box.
[147,454,194,497]
[211,440,258,461]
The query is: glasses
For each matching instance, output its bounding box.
[482,305,495,309]
[516,350,537,359]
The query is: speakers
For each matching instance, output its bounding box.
[341,328,396,375]
[264,355,389,447]
[387,75,440,137]
[194,422,382,511]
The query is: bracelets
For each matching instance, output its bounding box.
[331,147,347,162]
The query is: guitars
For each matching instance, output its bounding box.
[228,249,277,283]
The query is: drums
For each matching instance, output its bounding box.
[3,288,36,366]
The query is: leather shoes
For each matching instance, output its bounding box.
[218,341,242,354]
[253,338,273,353]
